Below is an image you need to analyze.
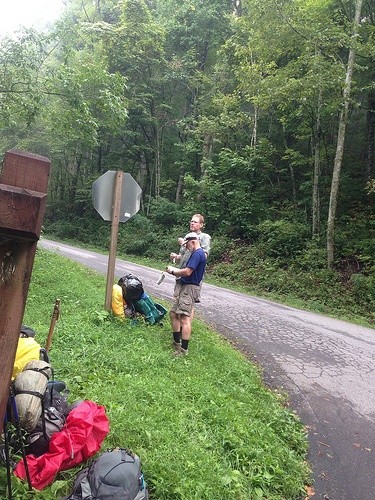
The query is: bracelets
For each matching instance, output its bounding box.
[172,270,176,276]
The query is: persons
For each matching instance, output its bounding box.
[165,232,207,358]
[169,214,211,342]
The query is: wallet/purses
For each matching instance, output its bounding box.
[157,274,165,285]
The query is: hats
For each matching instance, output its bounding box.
[181,231,200,246]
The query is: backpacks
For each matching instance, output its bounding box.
[134,292,168,326]
[112,284,125,319]
[7,324,110,500]
[62,447,148,500]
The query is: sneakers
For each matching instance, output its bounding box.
[172,348,188,356]
[173,342,181,349]
